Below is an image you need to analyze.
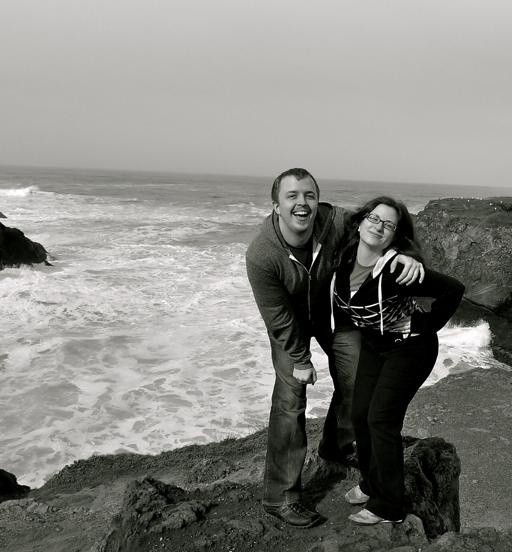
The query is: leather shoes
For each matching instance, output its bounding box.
[262,500,322,531]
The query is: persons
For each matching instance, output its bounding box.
[244,167,426,529]
[329,195,465,527]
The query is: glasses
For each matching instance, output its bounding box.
[363,213,398,233]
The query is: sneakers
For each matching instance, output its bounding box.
[347,508,403,525]
[317,444,359,467]
[344,484,369,505]
